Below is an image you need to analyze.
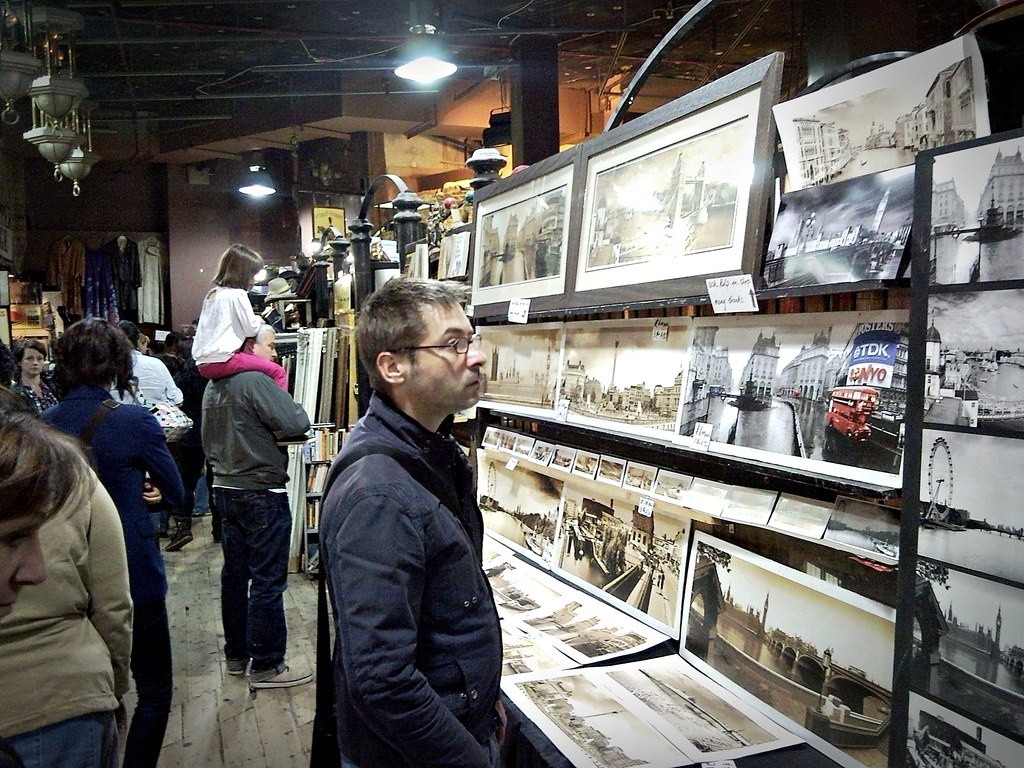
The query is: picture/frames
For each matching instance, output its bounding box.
[463,32,1023,768]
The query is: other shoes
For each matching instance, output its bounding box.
[226,657,251,674]
[249,667,315,687]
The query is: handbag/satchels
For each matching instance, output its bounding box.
[149,399,195,443]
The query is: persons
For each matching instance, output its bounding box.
[38,318,182,768]
[11,339,62,420]
[191,243,287,392]
[201,323,313,689]
[0,385,95,622]
[121,319,224,550]
[310,277,508,768]
[0,340,135,768]
[657,569,665,595]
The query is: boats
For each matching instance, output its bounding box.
[876,542,895,556]
[525,532,544,556]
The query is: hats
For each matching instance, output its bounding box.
[269,279,292,296]
[280,271,300,279]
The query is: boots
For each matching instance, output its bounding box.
[166,516,193,549]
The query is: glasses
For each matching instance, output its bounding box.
[385,336,482,356]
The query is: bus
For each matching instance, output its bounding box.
[827,386,878,443]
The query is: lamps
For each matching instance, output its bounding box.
[238,150,276,196]
[0,0,104,197]
[394,0,457,81]
